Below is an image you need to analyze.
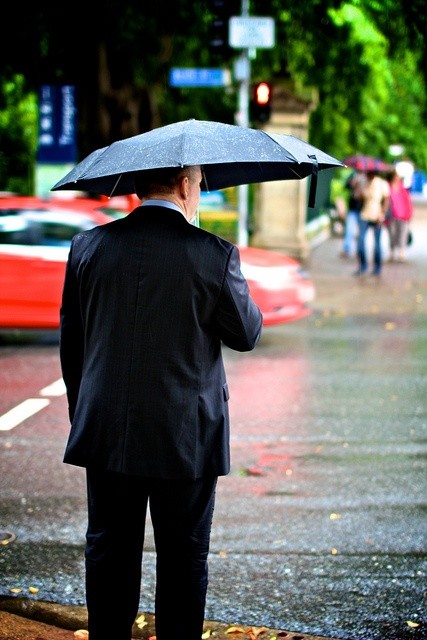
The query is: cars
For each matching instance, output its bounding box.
[0,194,315,348]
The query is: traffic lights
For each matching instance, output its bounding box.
[249,82,272,127]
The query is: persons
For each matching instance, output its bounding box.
[343,171,365,259]
[59,161,264,640]
[354,170,391,279]
[385,171,411,262]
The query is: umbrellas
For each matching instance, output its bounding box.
[341,153,394,173]
[51,117,347,194]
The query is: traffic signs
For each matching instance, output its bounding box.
[172,68,232,87]
[229,15,274,49]
